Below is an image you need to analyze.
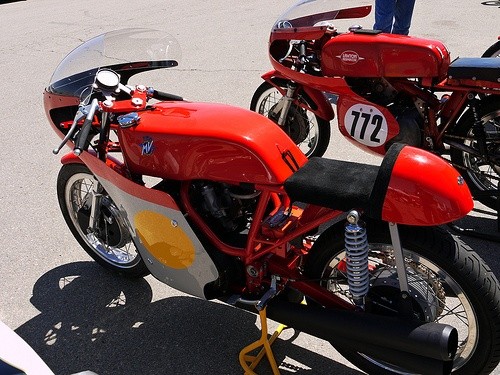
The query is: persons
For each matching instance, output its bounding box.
[373,0,416,36]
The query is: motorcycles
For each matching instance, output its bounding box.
[250,0,500,214]
[44,28,500,375]
